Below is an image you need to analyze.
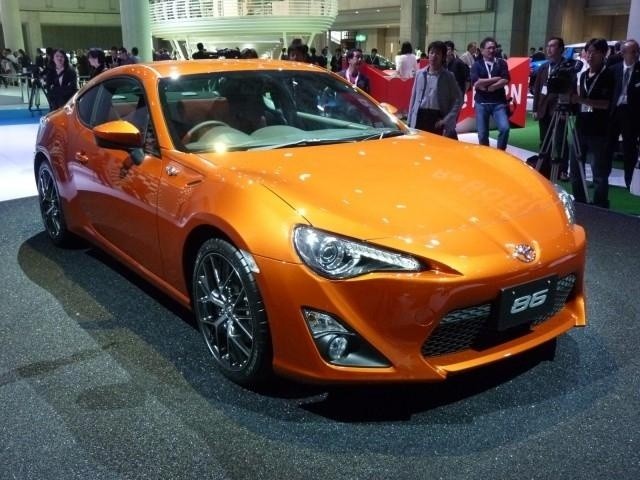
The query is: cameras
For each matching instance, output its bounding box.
[27,64,39,73]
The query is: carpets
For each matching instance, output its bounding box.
[2,193,640,479]
[1,95,54,126]
[485,113,640,218]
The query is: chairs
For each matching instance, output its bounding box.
[110,96,250,151]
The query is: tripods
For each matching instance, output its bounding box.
[28,79,48,110]
[534,104,590,204]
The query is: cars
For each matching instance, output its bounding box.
[528,41,621,93]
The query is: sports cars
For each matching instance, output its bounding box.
[31,58,593,392]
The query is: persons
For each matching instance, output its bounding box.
[570,38,619,210]
[530,47,546,62]
[153,48,176,61]
[394,39,508,139]
[1,47,141,110]
[281,38,380,125]
[470,38,510,151]
[531,36,578,183]
[192,44,259,94]
[607,39,640,192]
